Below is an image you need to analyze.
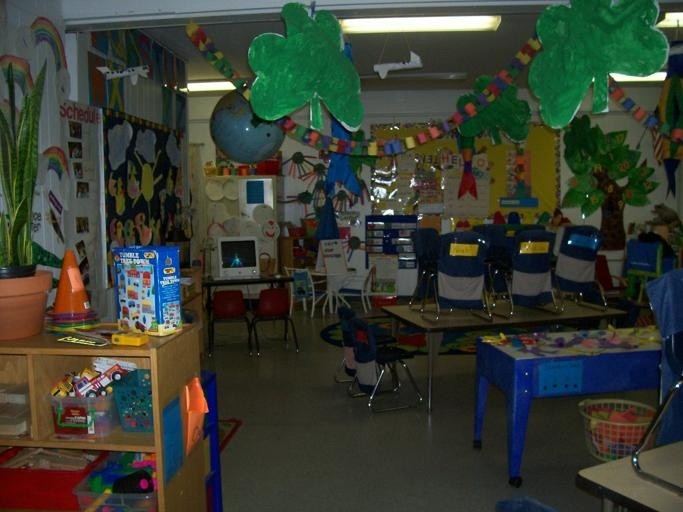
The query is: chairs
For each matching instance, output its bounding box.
[336,265,375,312]
[285,267,329,319]
[419,231,494,323]
[632,268,683,494]
[554,225,609,312]
[253,289,299,356]
[208,290,253,356]
[505,229,560,318]
[598,239,676,307]
[334,307,423,411]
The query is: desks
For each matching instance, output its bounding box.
[203,274,298,309]
[2,321,207,512]
[380,291,630,413]
[577,441,682,512]
[310,270,357,313]
[471,325,668,489]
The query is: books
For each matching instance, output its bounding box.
[0,385,31,439]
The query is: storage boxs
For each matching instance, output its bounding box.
[44,390,117,438]
[1,447,110,511]
[71,453,158,511]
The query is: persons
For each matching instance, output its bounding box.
[77,219,87,232]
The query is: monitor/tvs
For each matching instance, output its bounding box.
[211,236,260,279]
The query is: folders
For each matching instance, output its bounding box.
[367,222,417,268]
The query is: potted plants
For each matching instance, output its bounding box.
[1,61,54,339]
